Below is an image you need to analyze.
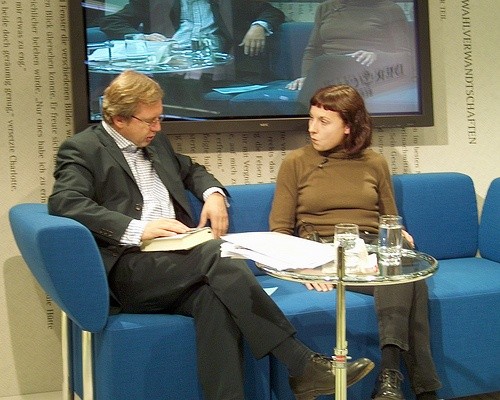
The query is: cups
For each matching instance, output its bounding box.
[333,223,359,267]
[377,214,402,266]
[124,34,148,63]
[191,32,213,64]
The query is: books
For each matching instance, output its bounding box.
[140,226,215,252]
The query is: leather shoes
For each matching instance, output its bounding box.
[288,352,375,400]
[373,369,406,400]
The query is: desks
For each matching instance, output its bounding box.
[256,244,439,400]
[89,51,233,76]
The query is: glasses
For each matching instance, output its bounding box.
[128,114,164,126]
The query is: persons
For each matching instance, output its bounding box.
[98,0,285,107]
[268,84,442,400]
[285,0,417,114]
[48,69,374,400]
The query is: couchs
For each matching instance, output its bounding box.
[202,22,418,116]
[8,171,500,400]
